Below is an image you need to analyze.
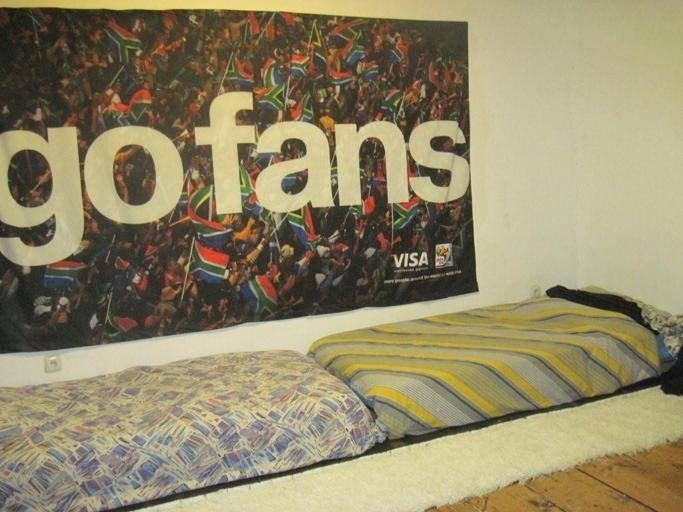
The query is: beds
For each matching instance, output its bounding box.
[307,286,683,441]
[1,349,387,512]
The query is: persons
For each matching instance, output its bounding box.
[1,7,472,351]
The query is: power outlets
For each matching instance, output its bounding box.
[45,355,61,374]
